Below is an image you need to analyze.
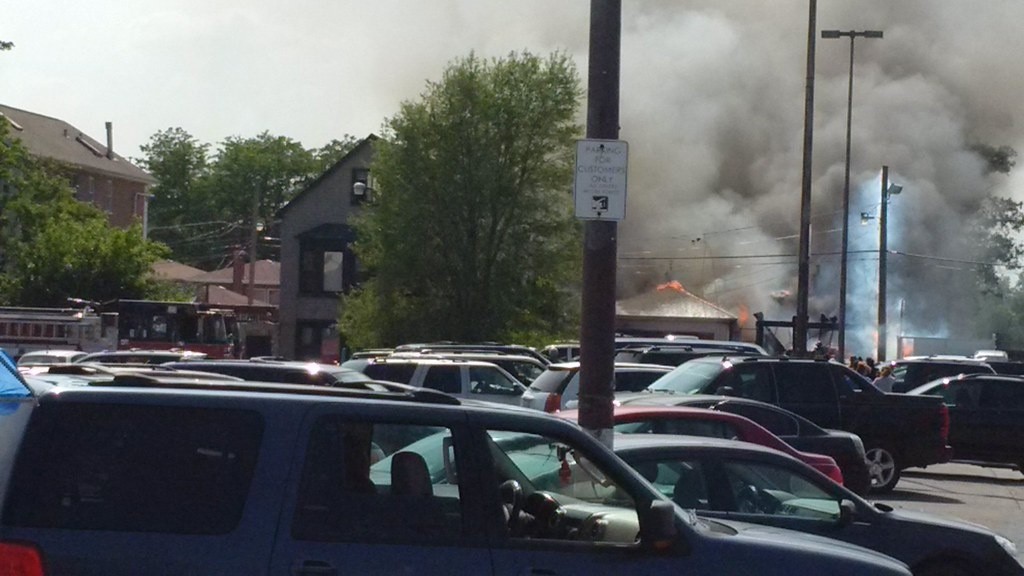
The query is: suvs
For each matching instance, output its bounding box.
[0,386,910,575]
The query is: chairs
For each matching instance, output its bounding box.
[632,460,659,483]
[673,469,709,510]
[389,452,461,545]
[343,446,389,531]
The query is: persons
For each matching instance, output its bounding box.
[814,341,896,393]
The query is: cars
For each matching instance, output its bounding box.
[433,433,1023,576]
[0,329,1024,489]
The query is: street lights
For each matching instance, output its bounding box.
[876,166,903,365]
[820,28,884,365]
